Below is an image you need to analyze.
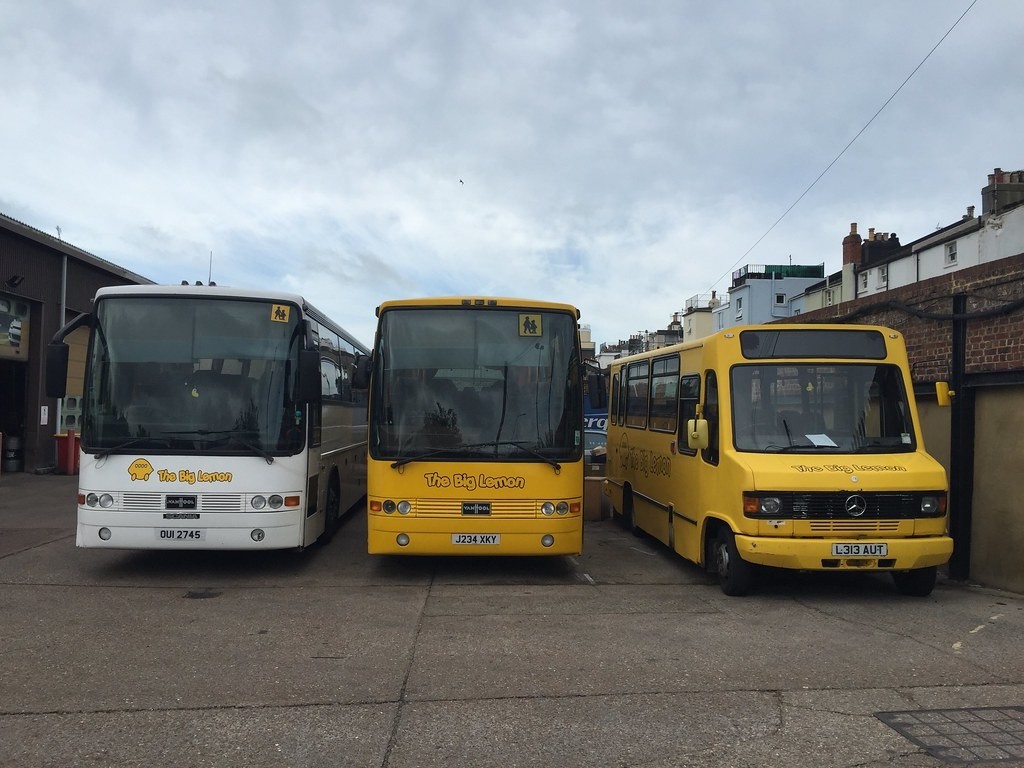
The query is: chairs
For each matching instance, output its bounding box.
[736,405,823,436]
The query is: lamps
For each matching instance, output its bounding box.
[5,274,24,287]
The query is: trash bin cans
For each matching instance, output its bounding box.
[53,433,81,475]
[584,476,610,522]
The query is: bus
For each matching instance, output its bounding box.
[605,323,955,596]
[45,280,374,554]
[352,296,609,565]
